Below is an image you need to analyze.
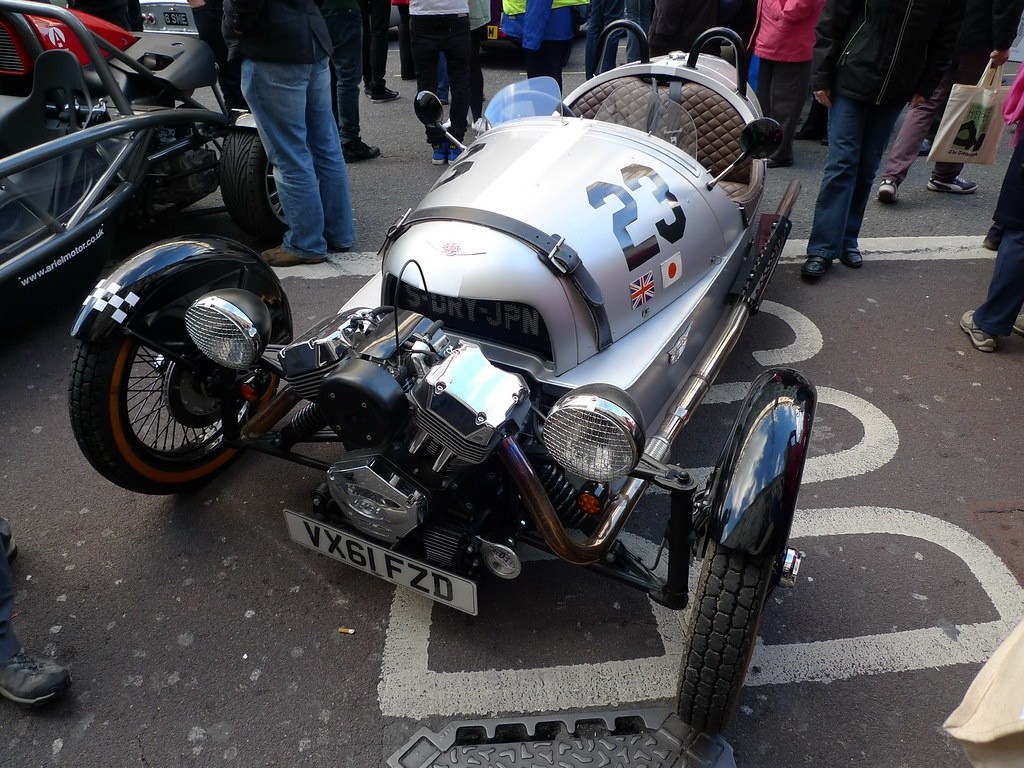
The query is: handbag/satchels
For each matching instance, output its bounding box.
[926,57,1012,165]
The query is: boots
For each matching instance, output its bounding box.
[369,79,400,103]
[363,78,372,99]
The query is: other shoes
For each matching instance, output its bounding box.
[1001,78,1008,86]
[767,158,794,167]
[441,100,448,105]
[442,118,451,128]
[431,142,449,164]
[820,137,829,145]
[918,139,933,156]
[983,227,1004,250]
[448,145,462,164]
[472,118,491,132]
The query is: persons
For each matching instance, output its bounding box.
[221,0,354,267]
[878,0,1024,202]
[960,127,1024,351]
[983,58,1024,250]
[801,0,967,275]
[0,515,72,707]
[188,0,829,165]
[66,0,144,32]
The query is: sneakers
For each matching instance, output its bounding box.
[838,247,862,268]
[960,310,998,352]
[343,136,380,162]
[6,534,17,566]
[801,255,832,278]
[877,179,900,202]
[927,175,979,194]
[326,242,352,252]
[261,246,327,267]
[0,648,72,704]
[1012,314,1024,336]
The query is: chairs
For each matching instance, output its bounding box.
[561,74,765,226]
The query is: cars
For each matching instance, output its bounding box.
[66,18,820,742]
[0,1,297,337]
